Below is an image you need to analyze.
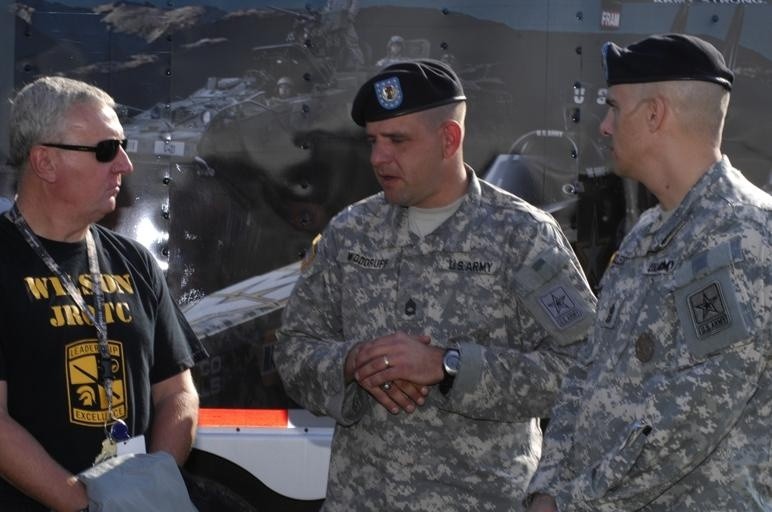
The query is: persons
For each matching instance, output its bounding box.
[376,36,409,67]
[0,76,210,512]
[520,32,772,512]
[276,77,295,98]
[272,58,598,512]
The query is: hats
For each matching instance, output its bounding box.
[605,33,734,93]
[350,59,467,126]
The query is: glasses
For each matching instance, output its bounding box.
[39,139,128,162]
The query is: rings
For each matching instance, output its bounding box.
[384,356,390,369]
[383,381,392,391]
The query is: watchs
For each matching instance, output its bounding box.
[439,348,460,394]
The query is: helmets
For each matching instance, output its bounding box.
[387,35,405,47]
[277,77,293,86]
